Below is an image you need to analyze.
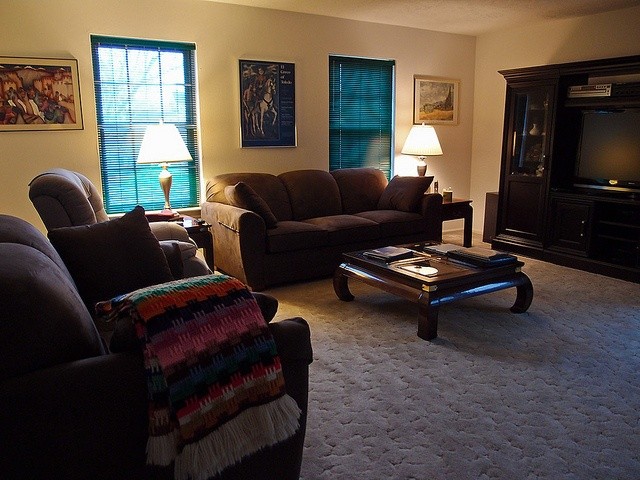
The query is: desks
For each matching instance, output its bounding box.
[180,213,215,274]
[426,194,473,247]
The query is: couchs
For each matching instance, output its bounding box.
[201,170,443,293]
[28,168,213,277]
[0,213,312,479]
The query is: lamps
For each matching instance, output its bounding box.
[136,120,193,209]
[401,122,443,155]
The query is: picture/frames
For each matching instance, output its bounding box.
[0,56,84,130]
[413,74,460,125]
[239,59,297,148]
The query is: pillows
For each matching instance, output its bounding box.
[48,205,174,318]
[377,175,434,211]
[224,182,278,228]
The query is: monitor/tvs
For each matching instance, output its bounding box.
[572,108,640,202]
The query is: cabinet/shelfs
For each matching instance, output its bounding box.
[492,54,640,285]
